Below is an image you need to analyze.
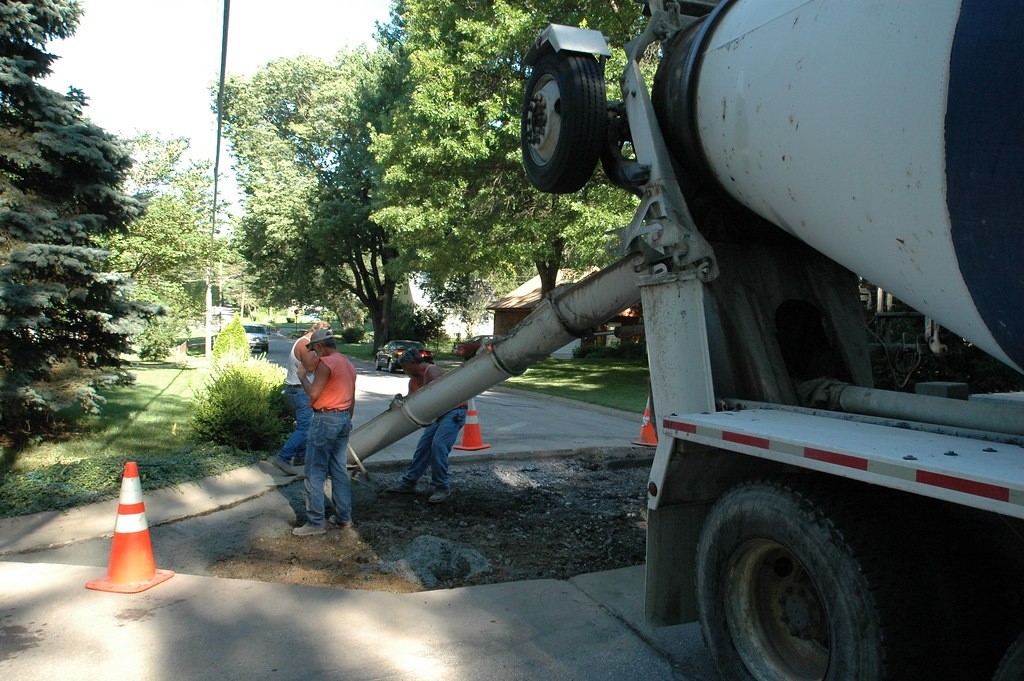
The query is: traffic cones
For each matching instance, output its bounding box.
[632,396,659,448]
[450,396,492,451]
[84,462,178,595]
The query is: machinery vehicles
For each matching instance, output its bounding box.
[520,0,1024,681]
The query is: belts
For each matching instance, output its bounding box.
[286,384,303,389]
[452,405,468,410]
[313,407,350,413]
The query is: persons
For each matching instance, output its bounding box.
[273,320,331,467]
[389,347,469,503]
[290,329,353,535]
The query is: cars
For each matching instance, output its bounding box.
[453,334,511,361]
[373,339,435,375]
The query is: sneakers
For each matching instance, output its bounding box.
[292,522,326,536]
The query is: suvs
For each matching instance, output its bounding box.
[241,324,270,353]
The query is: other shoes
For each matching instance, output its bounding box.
[272,455,295,476]
[428,484,451,503]
[293,455,305,466]
[387,476,417,493]
[329,515,353,527]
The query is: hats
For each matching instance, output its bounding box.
[305,329,334,351]
